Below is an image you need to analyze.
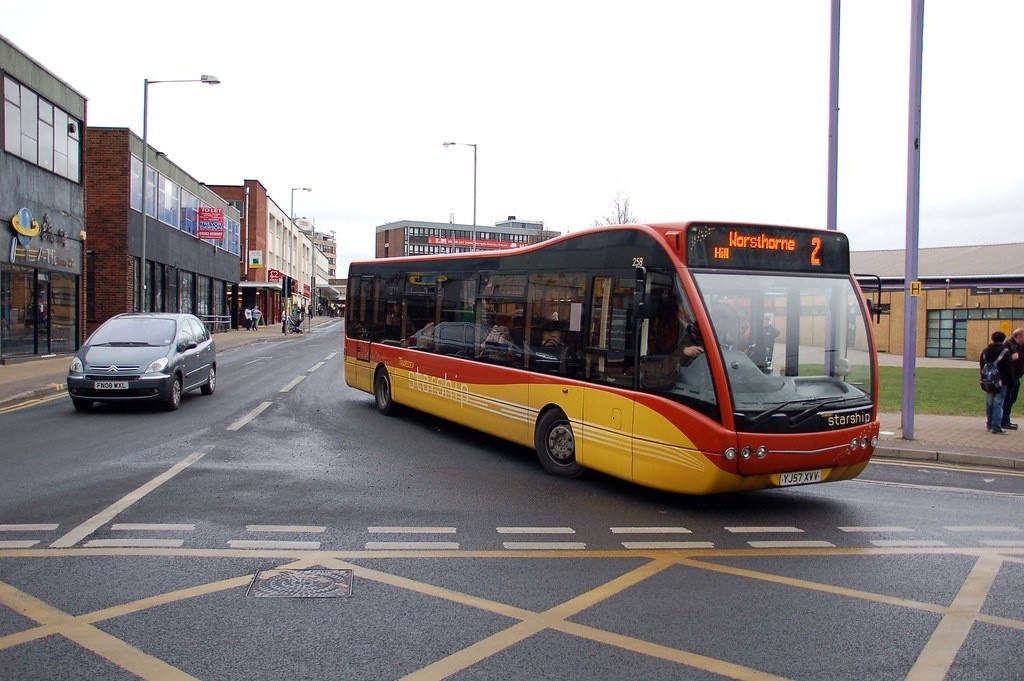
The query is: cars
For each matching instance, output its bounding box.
[408,320,582,375]
[67,313,217,412]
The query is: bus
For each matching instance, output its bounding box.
[343,219,884,497]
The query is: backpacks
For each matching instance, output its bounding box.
[978,348,1009,393]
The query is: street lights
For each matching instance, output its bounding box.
[448,212,456,253]
[139,72,222,314]
[443,140,477,253]
[291,187,314,316]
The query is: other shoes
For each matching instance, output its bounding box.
[991,428,1008,435]
[1001,423,1018,430]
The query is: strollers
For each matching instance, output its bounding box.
[286,314,305,335]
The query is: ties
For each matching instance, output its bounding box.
[686,324,702,346]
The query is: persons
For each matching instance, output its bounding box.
[996,328,1024,430]
[551,310,559,321]
[738,311,780,366]
[240,306,253,331]
[251,304,262,331]
[678,312,723,368]
[281,307,286,333]
[292,303,301,317]
[301,304,305,321]
[308,303,313,319]
[386,302,415,331]
[980,331,1012,435]
[480,315,517,362]
[326,302,344,318]
[318,300,324,316]
